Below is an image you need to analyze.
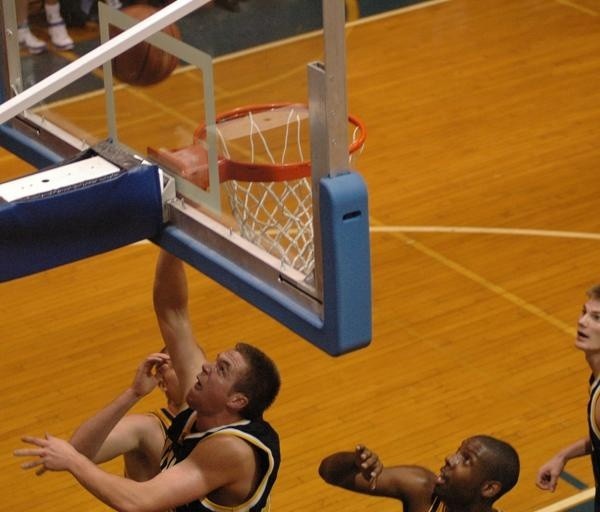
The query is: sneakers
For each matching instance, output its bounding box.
[17,29,45,55]
[47,20,75,52]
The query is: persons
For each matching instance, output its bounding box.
[68,342,205,512]
[535,283,600,512]
[318,435,520,512]
[11,246,282,512]
[15,0,124,54]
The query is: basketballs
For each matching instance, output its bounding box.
[110,5,179,84]
